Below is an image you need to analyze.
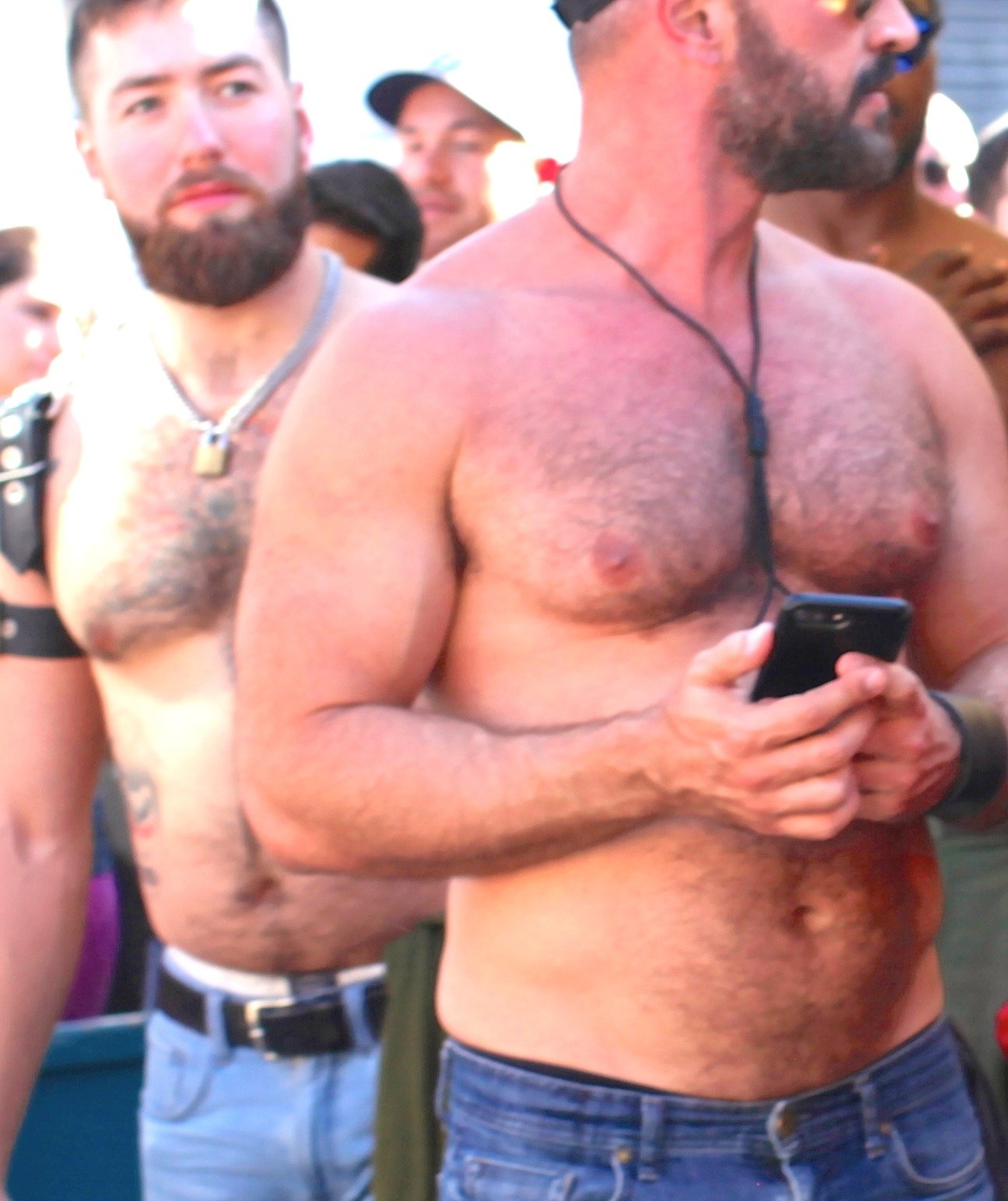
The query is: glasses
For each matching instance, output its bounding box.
[891,10,942,72]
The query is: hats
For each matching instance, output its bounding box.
[366,36,537,145]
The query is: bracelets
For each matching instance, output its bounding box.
[925,689,1008,823]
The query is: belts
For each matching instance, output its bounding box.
[156,968,389,1061]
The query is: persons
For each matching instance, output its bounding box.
[0,0,1008,1201]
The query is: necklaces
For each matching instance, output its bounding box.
[555,161,796,630]
[151,249,341,475]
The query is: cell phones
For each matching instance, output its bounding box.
[753,590,912,705]
